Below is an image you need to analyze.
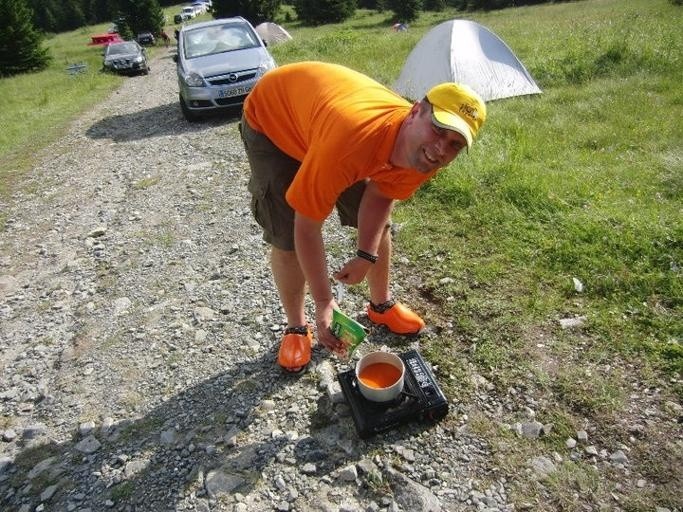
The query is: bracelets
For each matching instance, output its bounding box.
[357,249,378,264]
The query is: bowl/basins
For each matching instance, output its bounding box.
[352,351,405,403]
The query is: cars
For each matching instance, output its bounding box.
[173,15,278,122]
[132,32,155,46]
[102,40,148,75]
[181,2,209,19]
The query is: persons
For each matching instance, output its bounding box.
[160,29,168,39]
[240,62,488,377]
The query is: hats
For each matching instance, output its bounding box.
[426,81,488,156]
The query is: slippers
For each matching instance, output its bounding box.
[367,300,426,334]
[277,320,315,379]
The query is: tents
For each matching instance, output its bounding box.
[394,18,542,106]
[255,22,294,48]
